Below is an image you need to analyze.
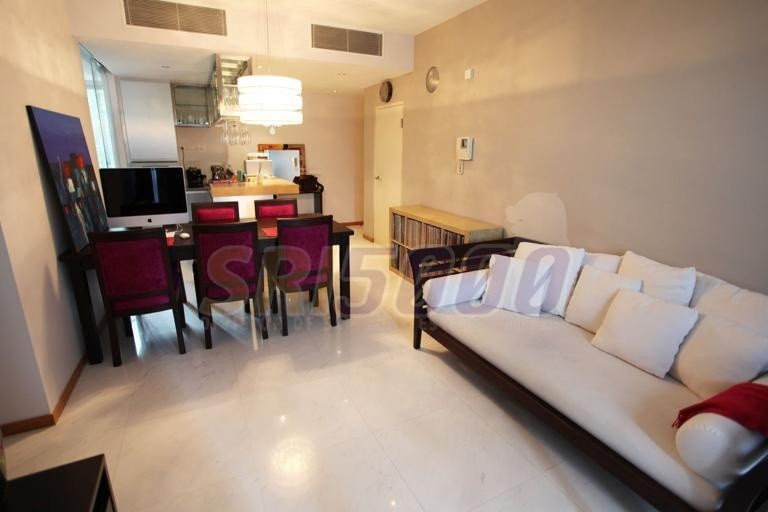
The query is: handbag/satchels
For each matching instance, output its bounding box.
[293,175,325,194]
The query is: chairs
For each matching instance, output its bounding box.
[263,216,338,336]
[86,229,186,367]
[191,221,267,349]
[254,198,315,302]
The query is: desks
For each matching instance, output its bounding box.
[190,201,252,314]
[58,212,353,365]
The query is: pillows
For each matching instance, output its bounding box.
[480,252,548,318]
[563,266,644,332]
[616,249,696,307]
[669,311,767,396]
[515,240,586,317]
[590,289,699,379]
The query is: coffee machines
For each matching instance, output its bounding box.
[186,166,206,189]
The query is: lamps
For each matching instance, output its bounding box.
[236,0,303,135]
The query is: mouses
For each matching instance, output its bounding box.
[178,232,191,239]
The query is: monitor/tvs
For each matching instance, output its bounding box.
[99,166,190,230]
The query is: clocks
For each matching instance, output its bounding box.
[379,80,392,102]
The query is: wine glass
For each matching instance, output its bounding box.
[218,121,249,145]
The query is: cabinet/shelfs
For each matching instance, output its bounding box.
[205,54,253,128]
[173,84,213,128]
[389,206,504,283]
[0,449,120,512]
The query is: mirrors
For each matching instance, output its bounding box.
[258,143,306,182]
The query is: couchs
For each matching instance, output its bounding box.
[406,235,768,512]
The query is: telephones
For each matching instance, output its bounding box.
[456,136,474,162]
